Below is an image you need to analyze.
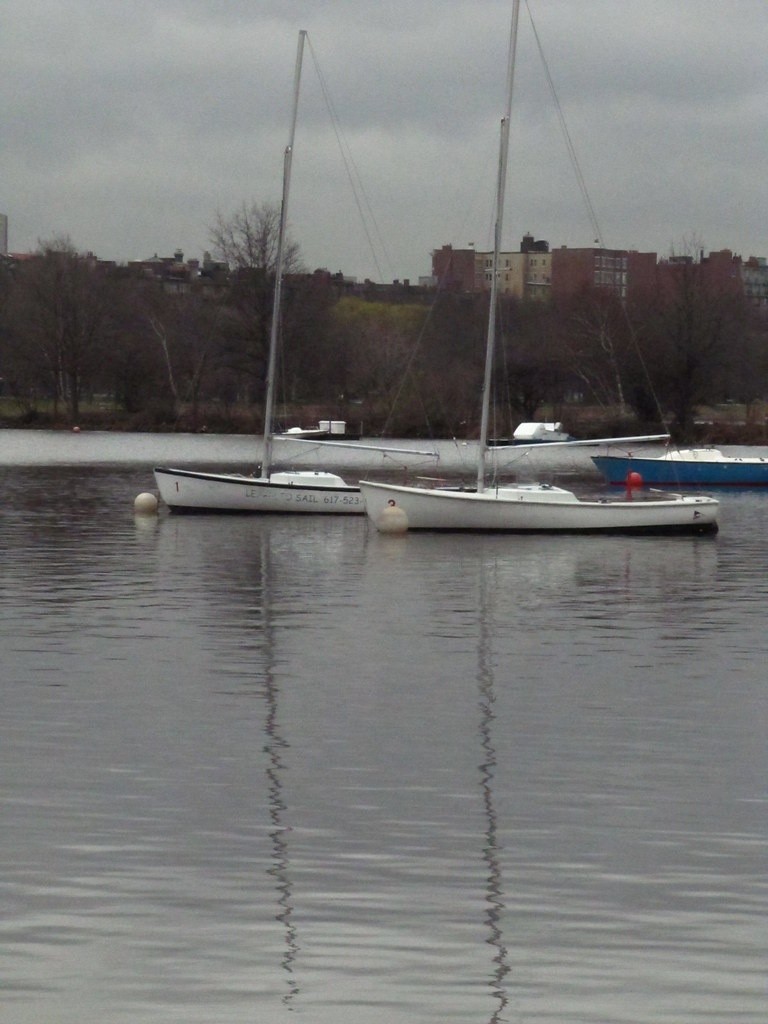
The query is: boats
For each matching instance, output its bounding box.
[280,420,362,442]
[488,421,577,445]
[587,447,766,489]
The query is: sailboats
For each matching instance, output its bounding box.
[360,0,724,540]
[126,27,377,513]
